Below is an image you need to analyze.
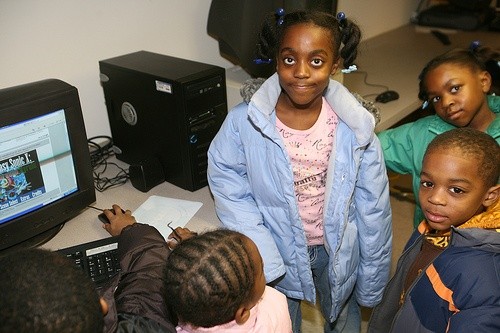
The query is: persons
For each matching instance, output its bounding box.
[207,7,393,333]
[163,226,294,333]
[375,40,500,230]
[365,126,500,333]
[0,204,177,333]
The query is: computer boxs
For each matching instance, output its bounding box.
[99,49,229,192]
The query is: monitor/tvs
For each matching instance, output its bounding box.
[0,78,96,253]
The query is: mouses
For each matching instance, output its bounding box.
[98,208,125,224]
[375,90,400,103]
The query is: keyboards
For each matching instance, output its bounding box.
[51,234,123,296]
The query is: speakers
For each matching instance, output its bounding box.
[129,158,165,192]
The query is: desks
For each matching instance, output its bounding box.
[341,24,500,132]
[37,140,222,250]
